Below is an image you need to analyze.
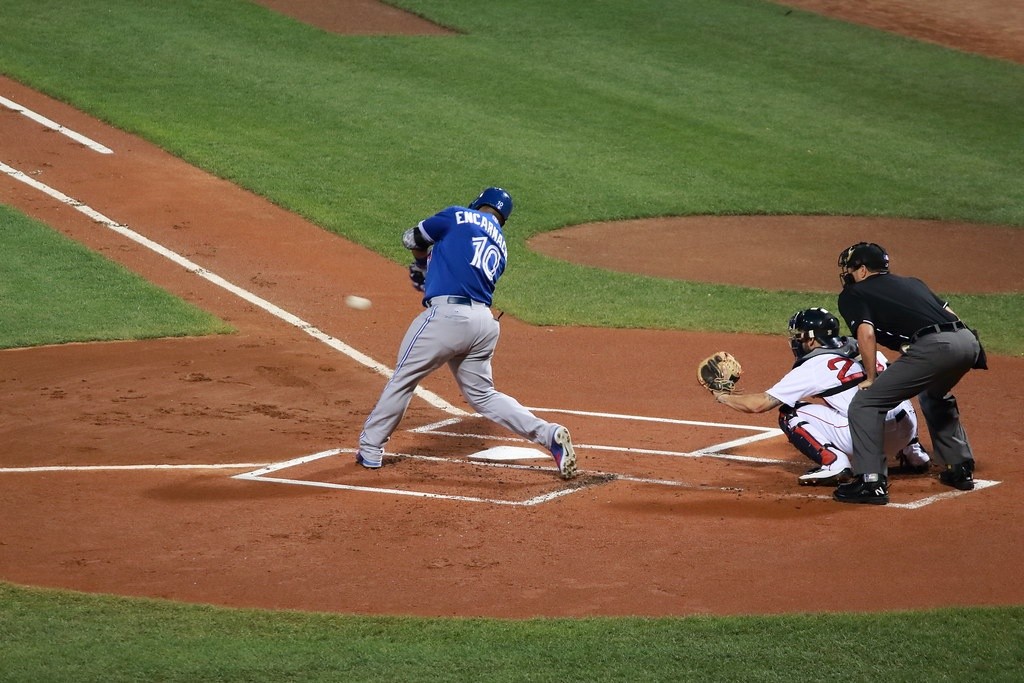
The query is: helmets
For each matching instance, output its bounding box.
[789,307,844,349]
[837,242,890,272]
[468,187,513,227]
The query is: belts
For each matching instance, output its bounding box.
[911,321,964,343]
[427,296,489,307]
[895,409,906,422]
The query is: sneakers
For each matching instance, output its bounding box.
[938,462,974,490]
[833,473,892,505]
[797,444,854,487]
[550,425,578,479]
[895,442,930,474]
[356,449,382,468]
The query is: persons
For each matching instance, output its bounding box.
[355,187,577,480]
[831,242,979,504]
[697,307,932,486]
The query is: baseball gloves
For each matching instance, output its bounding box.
[696,349,745,394]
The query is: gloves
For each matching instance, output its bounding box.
[408,259,427,292]
